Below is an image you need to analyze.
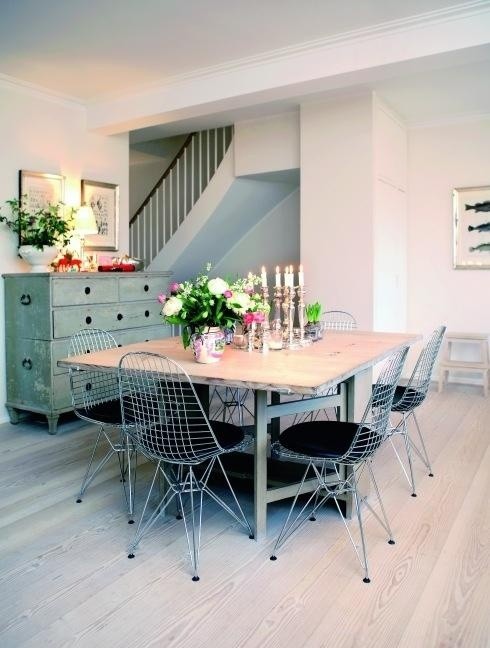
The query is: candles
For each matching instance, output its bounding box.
[276,266,281,286]
[284,267,288,286]
[289,265,295,287]
[299,265,303,285]
[262,267,267,288]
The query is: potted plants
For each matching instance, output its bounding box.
[0,194,76,273]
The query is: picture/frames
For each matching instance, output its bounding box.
[81,179,119,252]
[452,184,490,271]
[18,170,66,257]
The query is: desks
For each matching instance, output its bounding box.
[57,328,422,540]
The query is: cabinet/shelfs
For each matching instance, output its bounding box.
[2,270,174,435]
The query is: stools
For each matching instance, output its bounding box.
[437,331,489,397]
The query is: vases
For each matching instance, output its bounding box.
[192,326,225,364]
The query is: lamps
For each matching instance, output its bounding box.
[74,207,98,272]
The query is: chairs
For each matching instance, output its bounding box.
[119,352,254,582]
[288,311,358,424]
[270,342,410,584]
[67,329,177,524]
[372,325,447,497]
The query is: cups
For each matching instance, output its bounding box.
[87,254,97,272]
[233,335,245,346]
[253,329,320,354]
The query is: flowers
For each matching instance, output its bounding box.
[160,276,266,346]
